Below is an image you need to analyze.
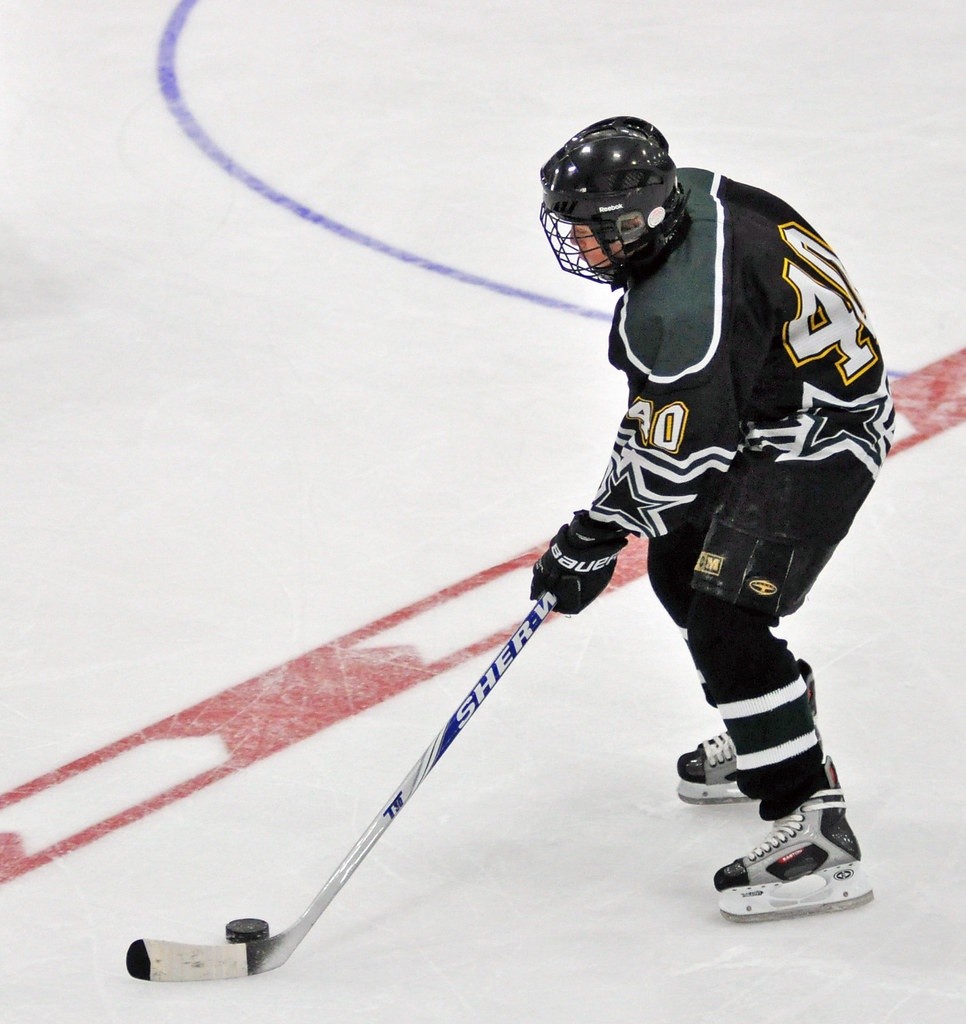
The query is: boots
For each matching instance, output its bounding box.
[677,659,822,804]
[714,756,874,922]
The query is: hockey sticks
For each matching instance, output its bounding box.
[125,591,562,982]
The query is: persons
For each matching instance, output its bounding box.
[525,113,898,925]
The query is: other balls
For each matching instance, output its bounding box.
[225,918,270,943]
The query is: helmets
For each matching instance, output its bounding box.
[540,116,691,247]
